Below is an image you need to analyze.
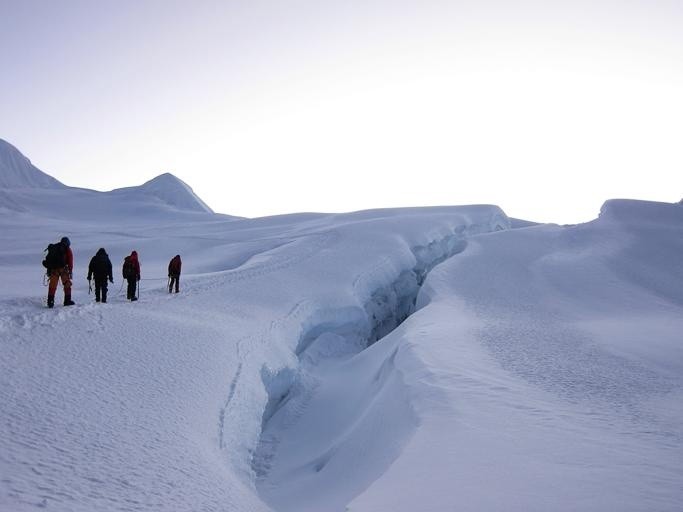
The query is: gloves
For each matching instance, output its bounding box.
[47,271,51,277]
[87,277,92,280]
[69,272,74,279]
[109,280,114,283]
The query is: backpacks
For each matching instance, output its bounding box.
[122,258,135,278]
[90,255,108,272]
[41,242,67,268]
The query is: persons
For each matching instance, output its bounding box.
[45,236,76,307]
[87,248,113,305]
[167,255,182,293]
[123,251,141,301]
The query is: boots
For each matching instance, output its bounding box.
[63,295,75,306]
[95,292,107,302]
[47,295,55,308]
[127,291,137,301]
